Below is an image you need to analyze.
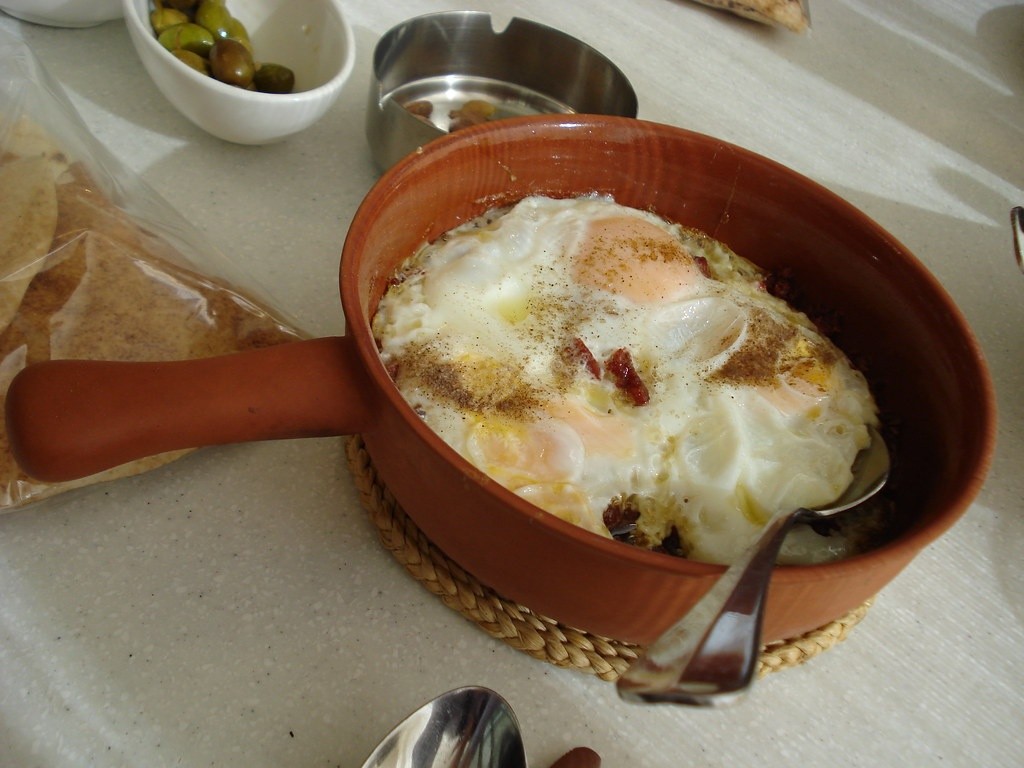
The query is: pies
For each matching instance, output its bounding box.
[695,0,810,36]
[0,130,316,513]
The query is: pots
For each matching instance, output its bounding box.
[6,114,997,643]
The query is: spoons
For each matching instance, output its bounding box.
[361,686,526,768]
[616,428,888,711]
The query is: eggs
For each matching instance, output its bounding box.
[369,191,878,568]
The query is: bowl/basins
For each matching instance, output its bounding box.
[123,0,356,145]
[0,0,122,26]
[367,10,637,182]
